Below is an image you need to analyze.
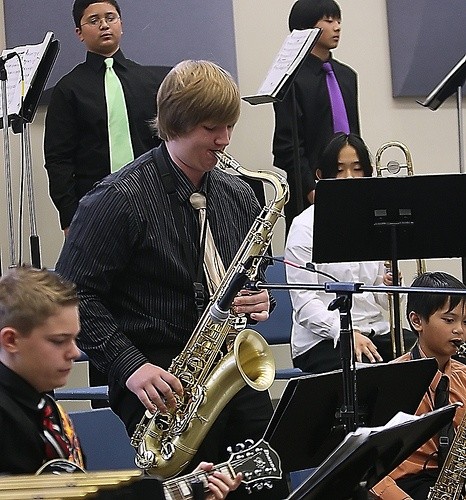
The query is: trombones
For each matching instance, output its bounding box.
[376,141,426,359]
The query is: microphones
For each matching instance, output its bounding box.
[0,52,17,65]
[209,254,255,323]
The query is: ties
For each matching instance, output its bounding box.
[37,393,85,468]
[103,58,135,177]
[190,191,236,351]
[323,61,349,133]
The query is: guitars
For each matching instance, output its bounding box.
[94,438,283,500]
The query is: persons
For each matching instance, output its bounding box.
[43,0,172,364]
[272,0,360,248]
[282,132,419,374]
[53,57,289,500]
[0,264,243,500]
[368,272,466,500]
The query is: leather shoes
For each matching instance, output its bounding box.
[434,376,454,472]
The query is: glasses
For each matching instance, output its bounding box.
[79,15,121,25]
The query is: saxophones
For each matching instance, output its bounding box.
[427,338,466,500]
[130,150,290,481]
